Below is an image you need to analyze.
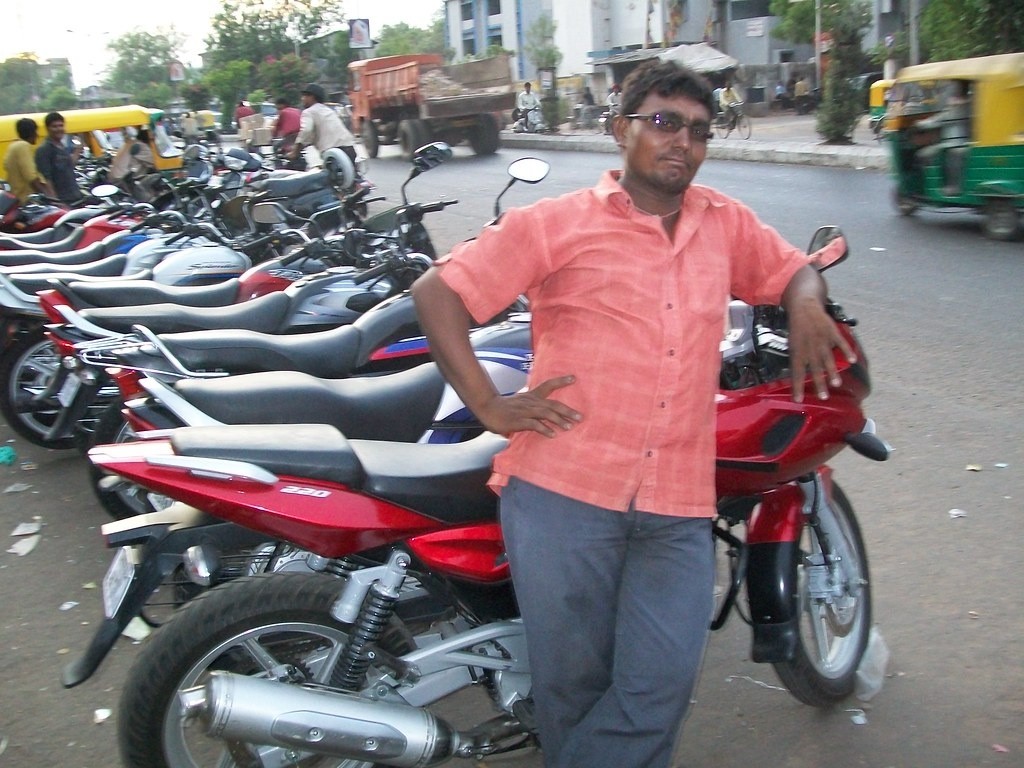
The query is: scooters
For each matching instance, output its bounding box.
[772,85,823,115]
[598,104,624,135]
[512,102,554,135]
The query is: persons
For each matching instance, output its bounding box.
[603,84,623,134]
[912,84,969,161]
[583,86,594,106]
[713,84,721,111]
[33,112,83,202]
[236,102,253,129]
[271,98,303,167]
[3,117,46,202]
[169,111,198,144]
[518,82,541,128]
[108,129,153,187]
[287,84,357,168]
[775,76,809,113]
[719,81,741,129]
[414,58,856,768]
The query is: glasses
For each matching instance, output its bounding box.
[623,111,714,144]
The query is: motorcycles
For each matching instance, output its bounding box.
[867,52,1023,242]
[60,222,893,767]
[152,101,281,142]
[1,136,554,677]
[1,104,188,201]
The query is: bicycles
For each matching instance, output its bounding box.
[715,101,753,140]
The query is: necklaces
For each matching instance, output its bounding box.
[635,206,679,218]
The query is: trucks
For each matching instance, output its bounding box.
[345,52,519,161]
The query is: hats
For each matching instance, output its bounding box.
[300,83,328,103]
